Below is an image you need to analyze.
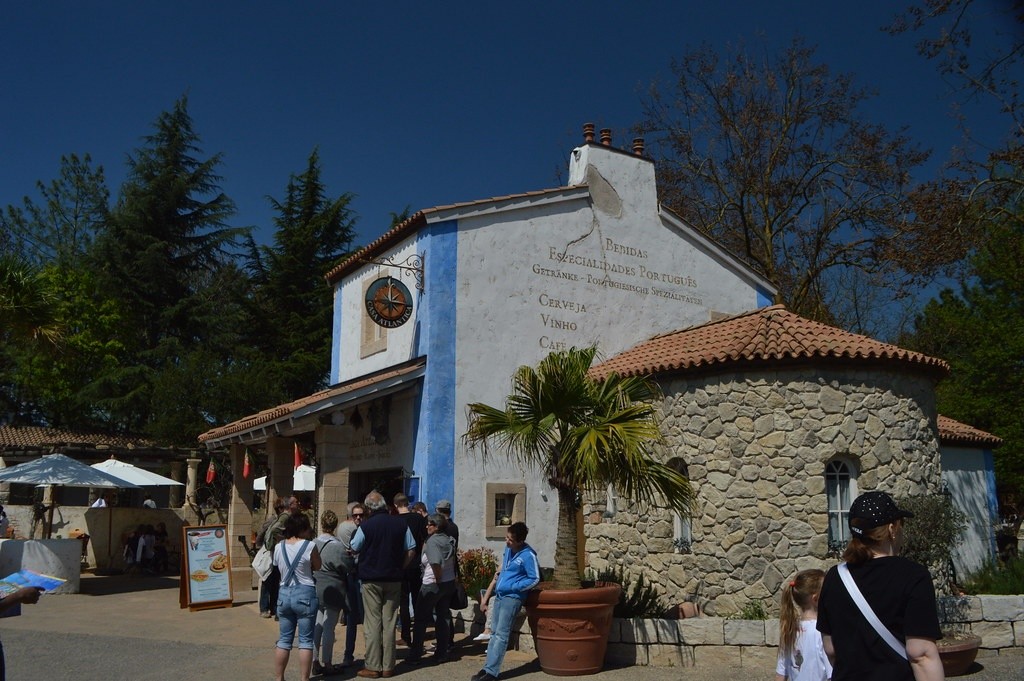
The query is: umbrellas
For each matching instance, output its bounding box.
[0,449,145,540]
[89,454,185,569]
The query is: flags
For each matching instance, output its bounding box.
[295,442,305,470]
[243,448,254,479]
[206,457,218,484]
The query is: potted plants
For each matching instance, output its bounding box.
[892,487,982,674]
[459,342,702,677]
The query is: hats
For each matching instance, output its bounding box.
[434,500,451,509]
[847,492,914,531]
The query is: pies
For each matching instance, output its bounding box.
[210,554,227,572]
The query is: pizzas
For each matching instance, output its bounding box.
[191,570,208,582]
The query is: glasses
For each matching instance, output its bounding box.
[351,513,364,519]
[427,522,436,527]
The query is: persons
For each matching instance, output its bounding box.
[255,495,286,618]
[473,563,503,653]
[312,510,357,675]
[0,505,9,538]
[349,488,459,678]
[273,512,322,681]
[264,492,301,621]
[471,521,542,681]
[127,522,170,577]
[774,569,833,681]
[337,502,360,548]
[143,495,156,508]
[338,504,365,668]
[816,490,946,681]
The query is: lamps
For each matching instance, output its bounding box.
[933,479,953,505]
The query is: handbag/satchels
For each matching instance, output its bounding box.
[449,582,468,610]
[251,544,274,581]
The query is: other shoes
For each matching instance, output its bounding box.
[472,632,490,641]
[382,669,394,677]
[429,656,447,662]
[485,649,487,653]
[426,646,451,653]
[396,638,411,646]
[404,657,420,665]
[470,669,496,681]
[324,666,340,676]
[343,655,355,666]
[430,642,454,648]
[312,661,324,675]
[259,610,271,618]
[356,669,380,678]
[410,640,426,647]
